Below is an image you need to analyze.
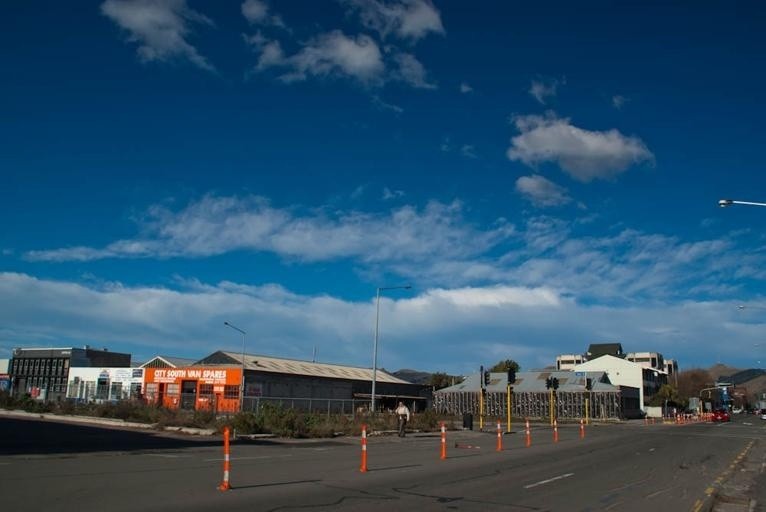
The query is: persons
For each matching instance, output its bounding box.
[395,401,410,437]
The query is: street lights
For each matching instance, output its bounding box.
[224,322,245,409]
[371,286,412,412]
[718,200,766,208]
[736,305,766,311]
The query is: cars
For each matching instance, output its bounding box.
[752,408,761,414]
[712,410,731,422]
[760,414,766,420]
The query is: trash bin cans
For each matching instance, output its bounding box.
[462,412,473,431]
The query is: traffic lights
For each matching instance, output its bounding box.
[485,372,490,386]
[510,387,513,394]
[545,377,559,389]
[508,369,515,384]
[586,379,591,390]
